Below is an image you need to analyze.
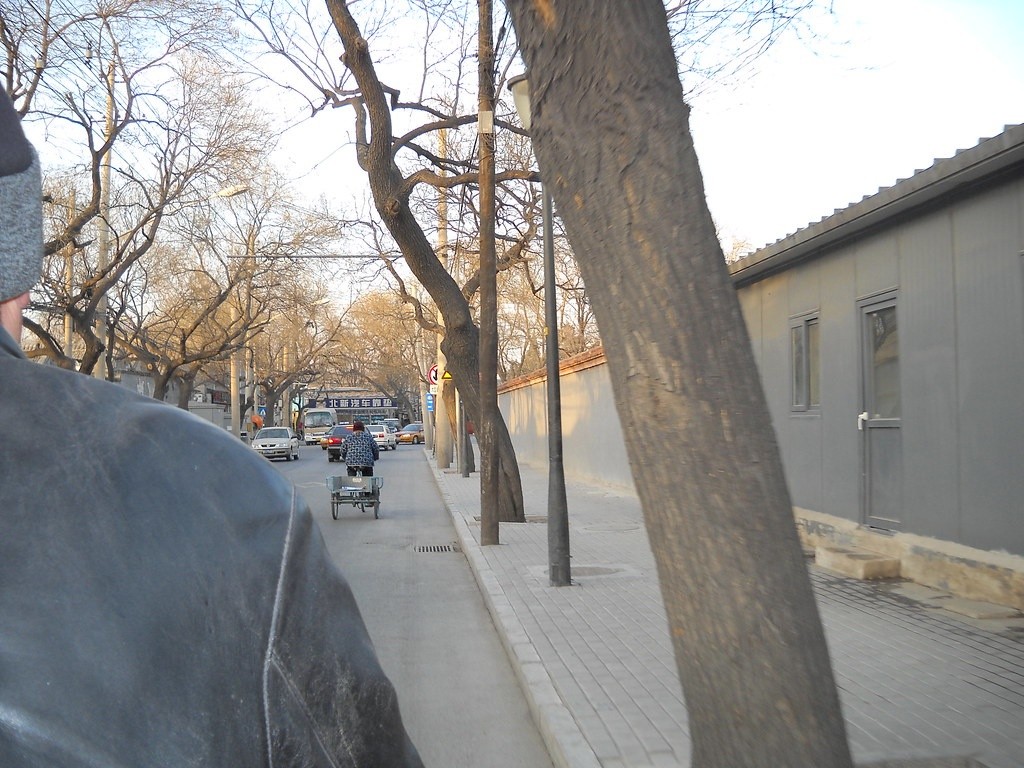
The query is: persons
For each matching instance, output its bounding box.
[339,421,380,506]
[0,81,426,768]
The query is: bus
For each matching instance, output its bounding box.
[303,407,338,445]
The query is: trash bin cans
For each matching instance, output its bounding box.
[241,431,249,445]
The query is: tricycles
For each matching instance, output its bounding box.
[326,467,383,519]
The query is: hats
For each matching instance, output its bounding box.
[352,422,365,431]
[0,81,45,305]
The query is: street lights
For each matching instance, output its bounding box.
[505,70,572,587]
[95,61,248,380]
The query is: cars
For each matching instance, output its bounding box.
[248,426,302,461]
[321,427,336,450]
[365,425,397,450]
[377,418,402,433]
[395,421,424,445]
[327,425,373,462]
